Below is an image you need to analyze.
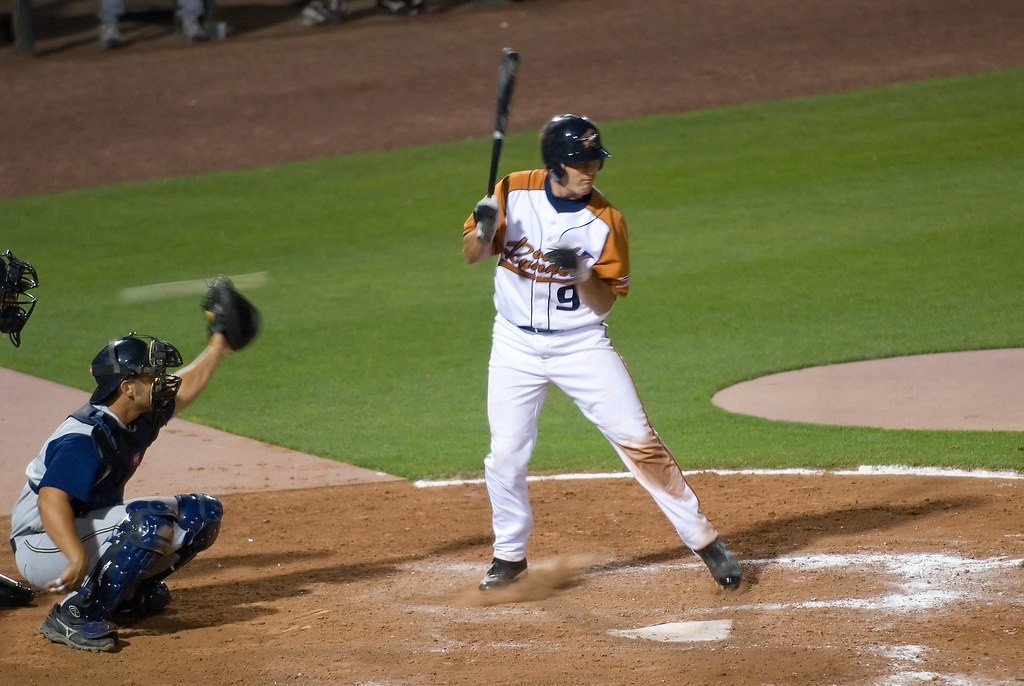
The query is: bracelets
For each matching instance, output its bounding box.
[576,267,590,281]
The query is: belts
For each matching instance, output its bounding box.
[520,326,551,334]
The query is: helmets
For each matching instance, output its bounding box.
[541,112,612,182]
[89,336,149,405]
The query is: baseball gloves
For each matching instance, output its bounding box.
[199,272,263,352]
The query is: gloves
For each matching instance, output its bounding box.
[473,196,498,244]
[542,248,590,283]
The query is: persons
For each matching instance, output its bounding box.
[98,1,425,48]
[0,250,264,652]
[464,115,744,592]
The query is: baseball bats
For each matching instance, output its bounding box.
[476,48,520,242]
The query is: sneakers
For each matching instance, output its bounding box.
[478,556,528,593]
[693,536,741,588]
[40,602,117,653]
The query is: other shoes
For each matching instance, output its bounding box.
[181,19,207,45]
[101,24,124,49]
